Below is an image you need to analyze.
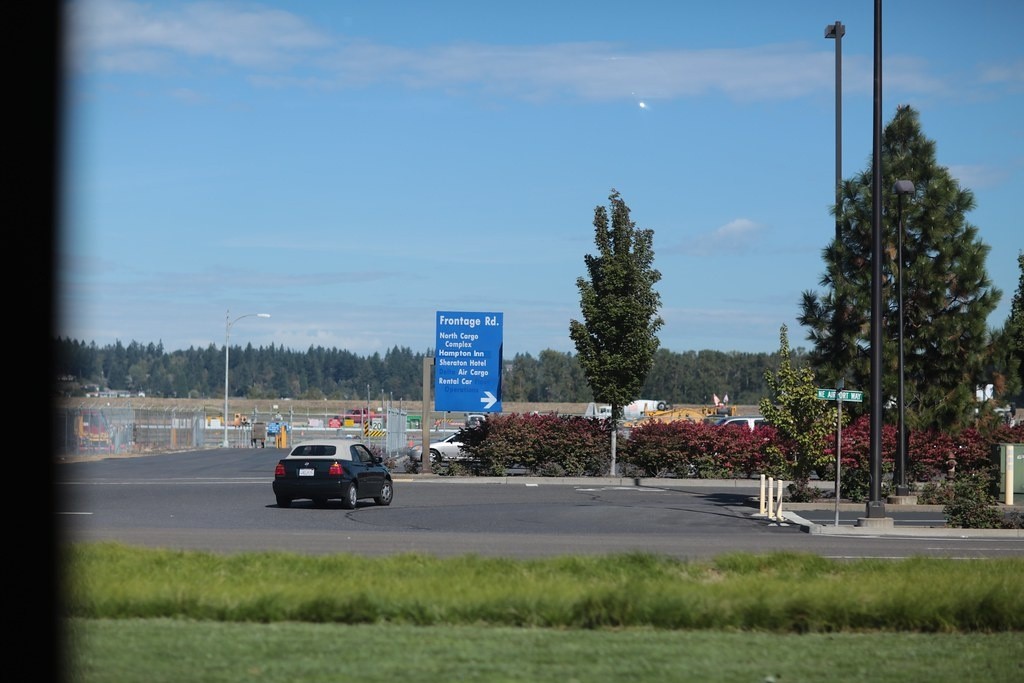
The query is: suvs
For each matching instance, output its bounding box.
[973,385,1017,432]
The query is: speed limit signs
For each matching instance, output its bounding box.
[372,418,382,430]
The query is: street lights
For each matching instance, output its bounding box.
[222,309,271,447]
[826,17,847,352]
[891,174,914,493]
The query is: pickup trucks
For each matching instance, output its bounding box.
[340,408,386,423]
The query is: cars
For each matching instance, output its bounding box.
[625,399,665,413]
[712,416,796,442]
[264,422,291,434]
[273,439,393,509]
[464,412,489,432]
[410,431,492,467]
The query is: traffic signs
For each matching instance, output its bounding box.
[434,309,502,414]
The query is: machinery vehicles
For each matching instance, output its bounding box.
[69,399,137,453]
[207,413,249,426]
[626,403,737,426]
